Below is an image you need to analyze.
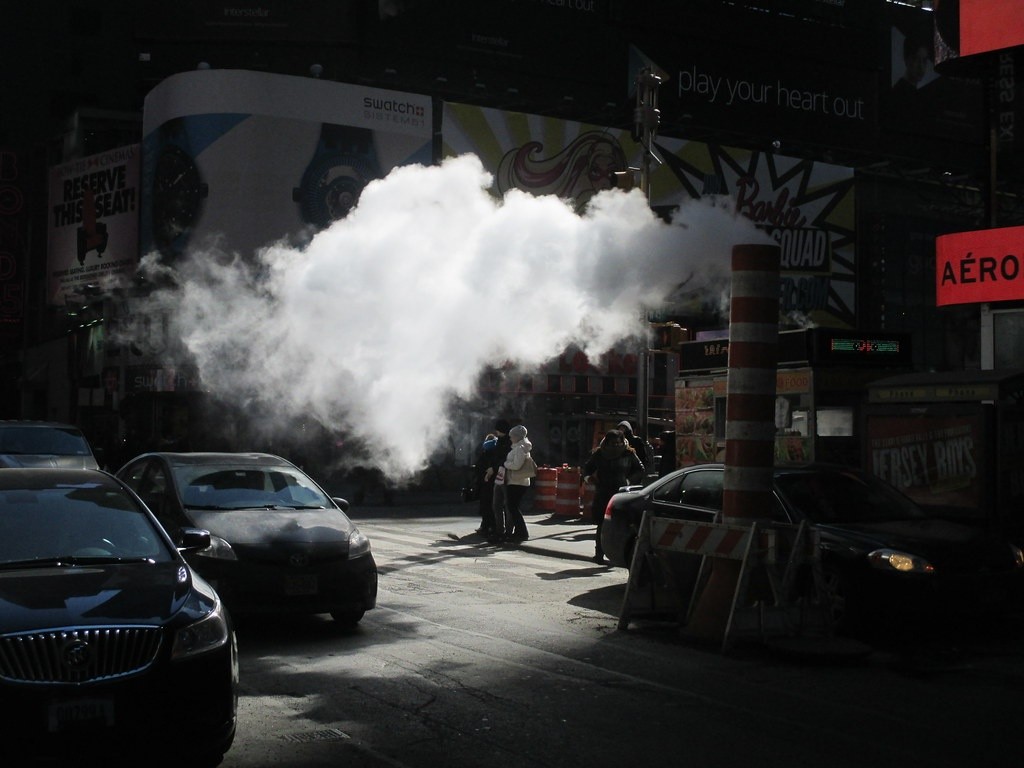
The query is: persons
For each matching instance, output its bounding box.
[485,421,511,538]
[83,366,137,473]
[475,426,498,536]
[504,425,532,541]
[582,431,646,561]
[617,421,646,486]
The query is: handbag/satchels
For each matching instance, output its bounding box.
[511,446,538,479]
[470,463,489,479]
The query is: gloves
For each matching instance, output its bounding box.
[588,477,597,485]
[615,481,626,490]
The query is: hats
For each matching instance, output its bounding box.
[509,426,528,438]
[495,420,510,434]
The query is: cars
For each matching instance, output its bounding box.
[600,465,1024,646]
[112,451,379,630]
[0,466,240,768]
[0,420,100,475]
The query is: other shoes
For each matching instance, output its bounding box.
[592,554,604,564]
[477,526,529,542]
